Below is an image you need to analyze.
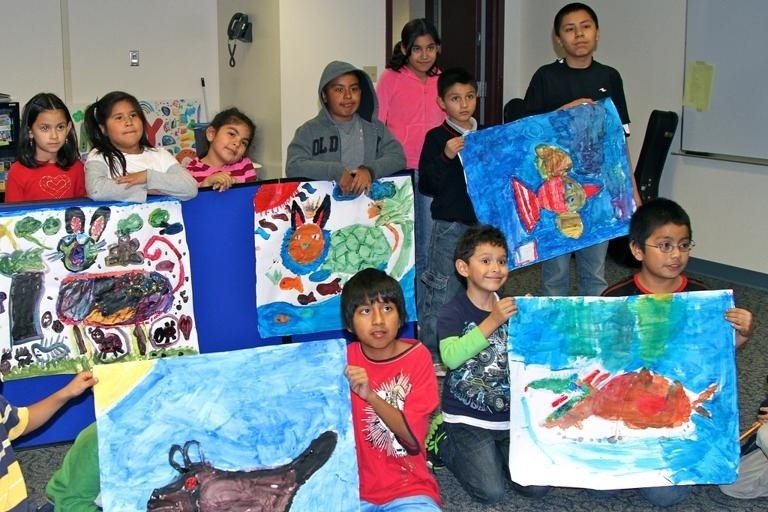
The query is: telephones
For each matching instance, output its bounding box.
[228,13,248,39]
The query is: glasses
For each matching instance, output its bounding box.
[645,240,696,253]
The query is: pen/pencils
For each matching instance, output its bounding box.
[739,422,762,442]
[685,150,711,156]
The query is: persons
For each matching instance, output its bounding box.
[81,92,199,205]
[1,367,100,512]
[4,93,87,206]
[40,422,100,512]
[416,67,493,376]
[284,61,405,196]
[178,108,257,196]
[718,396,766,498]
[422,223,549,503]
[372,17,450,172]
[586,199,751,506]
[523,3,644,296]
[342,269,445,512]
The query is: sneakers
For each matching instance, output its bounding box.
[424,407,447,474]
[433,363,447,376]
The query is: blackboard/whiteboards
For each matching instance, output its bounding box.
[680,0,768,165]
